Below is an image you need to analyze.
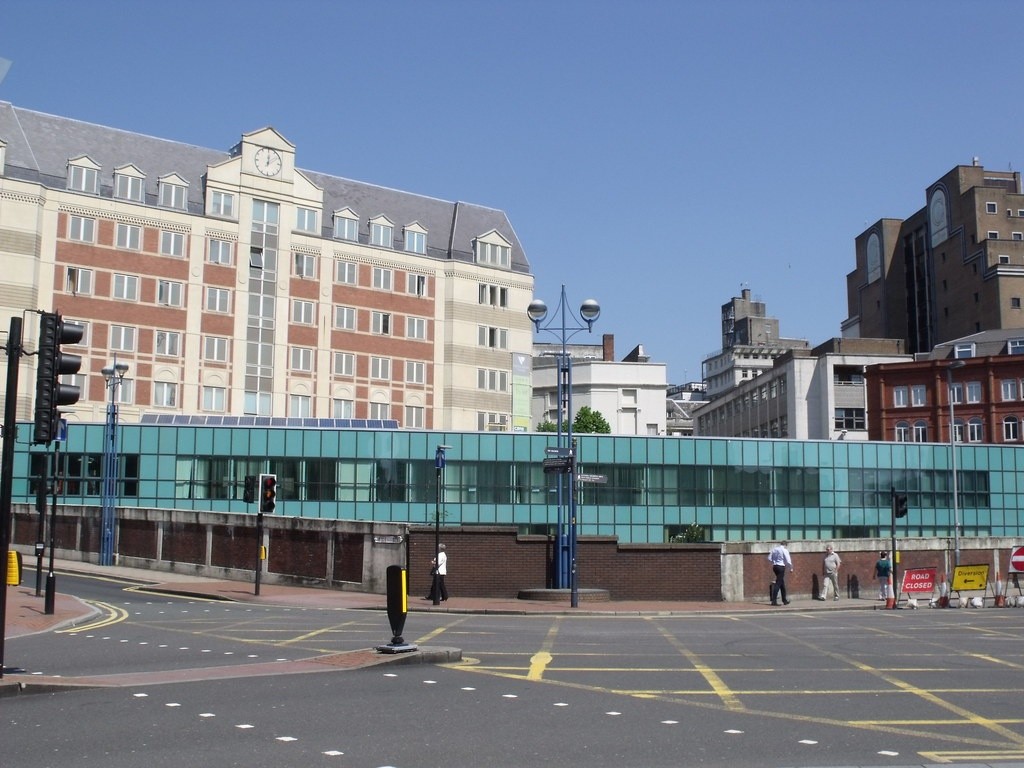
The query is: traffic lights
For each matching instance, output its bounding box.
[243,476,257,502]
[898,495,908,517]
[59,323,82,406]
[264,477,276,512]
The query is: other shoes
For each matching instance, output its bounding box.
[834,597,839,601]
[818,596,826,601]
[772,602,781,606]
[440,598,447,601]
[426,595,433,600]
[784,599,790,605]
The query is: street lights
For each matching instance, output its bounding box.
[434,445,453,605]
[101,353,128,564]
[947,361,968,568]
[527,284,600,589]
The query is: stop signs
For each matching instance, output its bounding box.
[1006,546,1024,572]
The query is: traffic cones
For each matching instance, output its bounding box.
[886,578,895,608]
[938,573,951,606]
[991,573,1005,605]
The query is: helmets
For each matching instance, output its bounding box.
[439,544,446,550]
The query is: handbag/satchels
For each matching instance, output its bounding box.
[430,567,434,575]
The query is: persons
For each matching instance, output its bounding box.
[421,543,448,601]
[767,540,794,606]
[818,545,843,601]
[873,551,891,600]
